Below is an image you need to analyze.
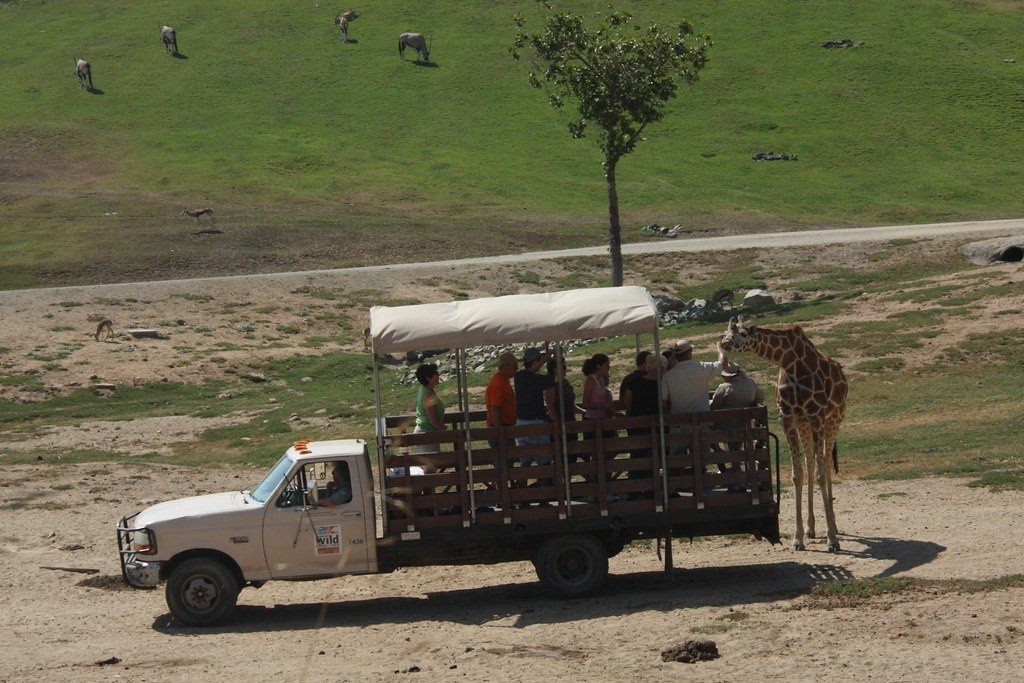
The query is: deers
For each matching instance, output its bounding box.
[93,319,115,340]
[181,207,215,232]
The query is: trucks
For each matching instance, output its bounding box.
[114,280,784,628]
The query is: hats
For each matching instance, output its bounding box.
[674,340,696,356]
[523,348,541,363]
[721,359,740,378]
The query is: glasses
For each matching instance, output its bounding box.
[332,470,340,476]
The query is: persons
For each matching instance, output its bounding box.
[710,362,764,491]
[317,461,352,505]
[485,346,579,509]
[619,340,728,497]
[413,364,455,512]
[582,353,624,503]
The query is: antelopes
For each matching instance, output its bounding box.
[73,51,93,91]
[398,33,432,63]
[157,18,178,55]
[335,9,359,42]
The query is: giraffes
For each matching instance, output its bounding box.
[719,315,848,552]
